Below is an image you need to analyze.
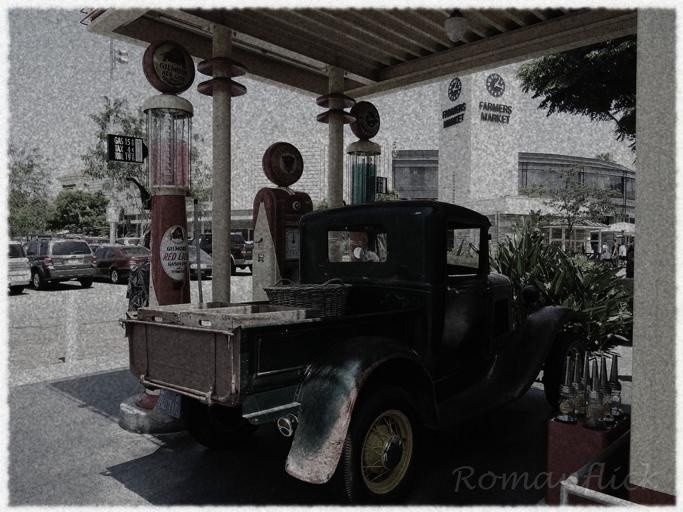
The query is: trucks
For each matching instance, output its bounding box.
[120,198,591,505]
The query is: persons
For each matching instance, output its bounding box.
[609,238,618,269]
[599,241,611,268]
[618,241,626,266]
[169,226,184,240]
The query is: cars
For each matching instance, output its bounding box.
[7,230,253,296]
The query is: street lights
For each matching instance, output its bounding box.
[107,36,128,247]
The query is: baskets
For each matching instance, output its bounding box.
[263,279,352,318]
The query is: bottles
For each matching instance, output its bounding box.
[557,350,626,429]
